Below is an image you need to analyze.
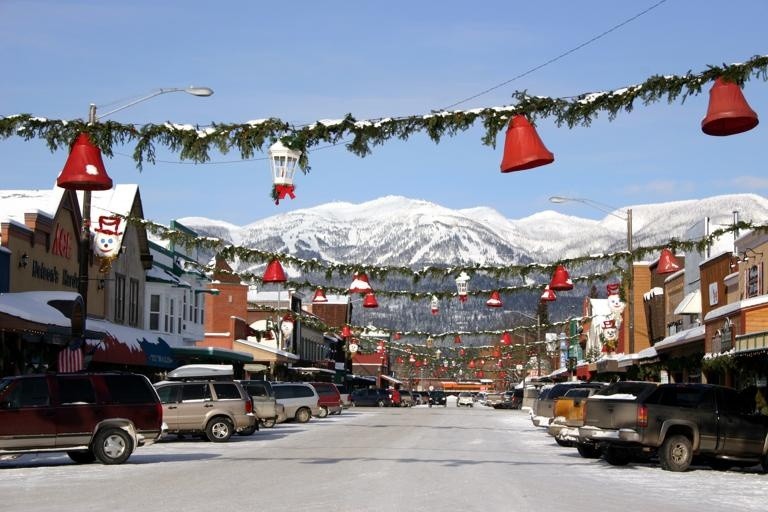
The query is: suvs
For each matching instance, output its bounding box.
[0,372,162,464]
[532,379,767,471]
[152,363,255,442]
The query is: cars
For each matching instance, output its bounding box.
[269,381,320,423]
[429,390,446,407]
[234,380,277,428]
[476,390,522,409]
[457,392,473,408]
[351,388,429,407]
[310,382,341,418]
[332,383,352,409]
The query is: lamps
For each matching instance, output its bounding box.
[98,280,106,291]
[729,247,764,268]
[17,253,29,269]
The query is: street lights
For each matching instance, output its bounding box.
[548,195,635,353]
[72,85,214,375]
